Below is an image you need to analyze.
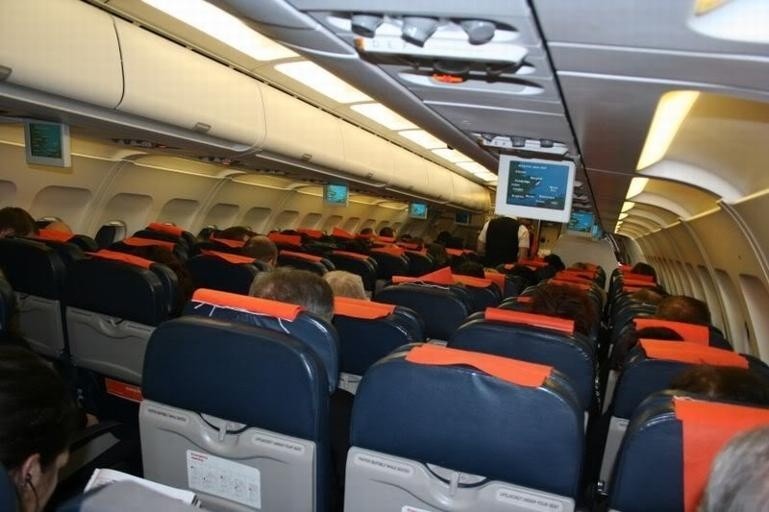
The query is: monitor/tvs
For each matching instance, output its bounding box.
[453,211,470,225]
[408,202,428,220]
[324,183,349,207]
[566,211,601,243]
[24,120,71,168]
[493,154,576,223]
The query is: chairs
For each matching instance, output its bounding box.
[1,216,769,509]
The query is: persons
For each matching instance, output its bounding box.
[0,208,768,511]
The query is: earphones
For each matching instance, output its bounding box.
[26,474,31,482]
